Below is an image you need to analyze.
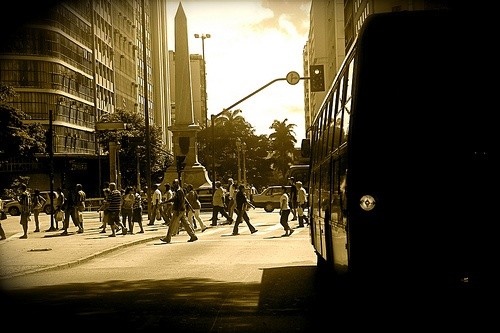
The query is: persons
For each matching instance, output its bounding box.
[233,184,258,234]
[210,181,255,225]
[288,177,297,220]
[0,224,6,240]
[279,186,295,236]
[99,183,208,237]
[55,184,86,236]
[17,183,31,239]
[295,181,310,228]
[160,179,198,243]
[32,190,46,232]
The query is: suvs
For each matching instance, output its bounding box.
[4,190,62,216]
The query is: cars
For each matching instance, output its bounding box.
[240,184,252,195]
[250,186,311,212]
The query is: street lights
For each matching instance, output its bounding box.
[115,140,121,193]
[241,141,247,188]
[135,144,141,192]
[194,33,211,130]
[235,136,241,187]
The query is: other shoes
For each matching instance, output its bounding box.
[251,230,258,234]
[297,225,304,228]
[291,219,297,221]
[281,234,288,237]
[188,237,198,242]
[96,223,145,238]
[289,230,294,236]
[1,226,87,240]
[231,233,240,235]
[146,218,234,233]
[159,238,170,243]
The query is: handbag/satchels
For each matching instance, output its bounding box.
[296,206,303,216]
[31,201,42,210]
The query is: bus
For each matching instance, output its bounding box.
[300,7,500,287]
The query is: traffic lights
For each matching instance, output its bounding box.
[44,132,52,155]
[176,155,187,174]
[310,64,325,92]
